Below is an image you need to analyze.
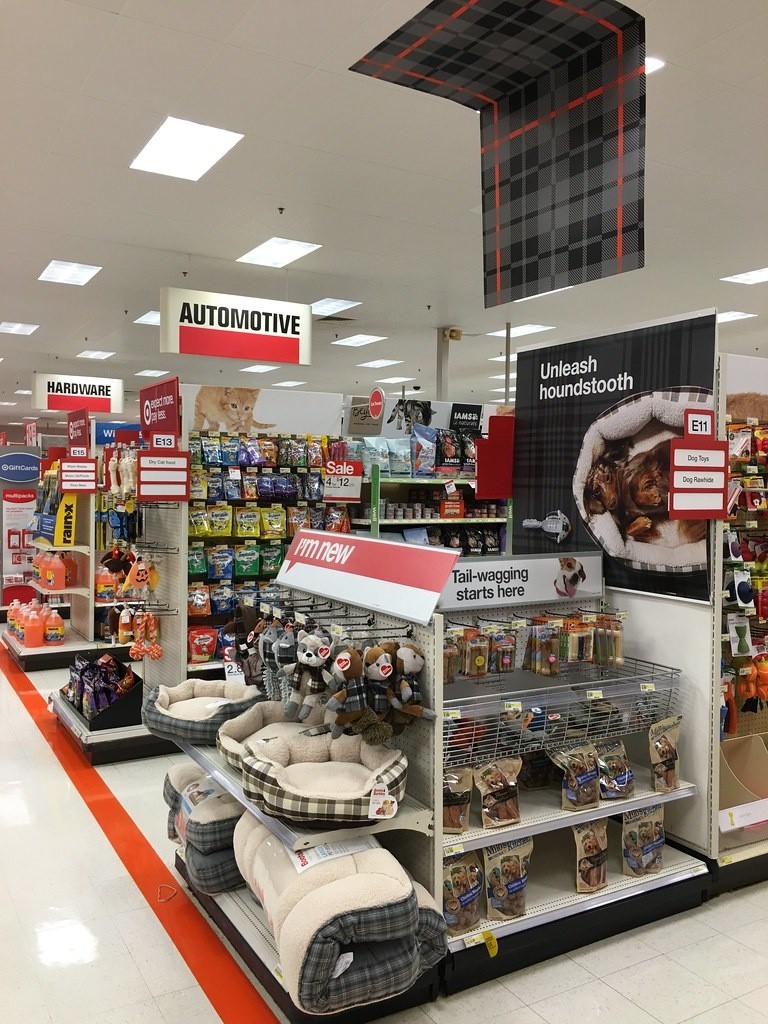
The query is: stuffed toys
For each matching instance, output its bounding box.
[243,614,437,746]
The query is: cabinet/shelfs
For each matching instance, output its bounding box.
[0,434,72,623]
[607,349,768,900]
[49,429,514,766]
[174,589,444,1024]
[2,419,143,672]
[436,598,713,997]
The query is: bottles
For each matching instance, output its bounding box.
[7,598,65,648]
[42,595,71,604]
[32,550,77,590]
[95,564,144,603]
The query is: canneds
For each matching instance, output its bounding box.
[347,487,507,520]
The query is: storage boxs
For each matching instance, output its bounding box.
[58,654,145,731]
[33,460,77,547]
[719,732,768,810]
